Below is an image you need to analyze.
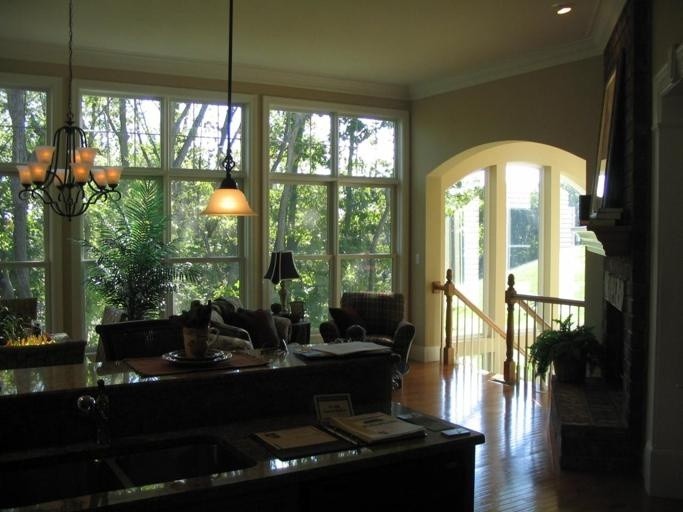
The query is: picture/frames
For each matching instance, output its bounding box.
[590,48,625,213]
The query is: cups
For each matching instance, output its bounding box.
[390,400,401,417]
[182,324,219,359]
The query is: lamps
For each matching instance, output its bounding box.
[264,250,301,315]
[16,0,122,222]
[201,0,257,216]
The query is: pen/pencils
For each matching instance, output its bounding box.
[323,425,358,445]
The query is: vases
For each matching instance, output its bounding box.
[183,326,206,361]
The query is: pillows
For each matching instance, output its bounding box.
[238,307,280,347]
[211,321,251,341]
[329,303,365,338]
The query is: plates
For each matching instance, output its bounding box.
[161,347,233,366]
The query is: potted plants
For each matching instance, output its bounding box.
[529,315,600,383]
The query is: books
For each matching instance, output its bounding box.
[591,208,624,227]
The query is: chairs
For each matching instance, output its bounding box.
[211,297,292,350]
[96,319,184,361]
[0,297,36,328]
[320,292,416,392]
[85,306,126,363]
